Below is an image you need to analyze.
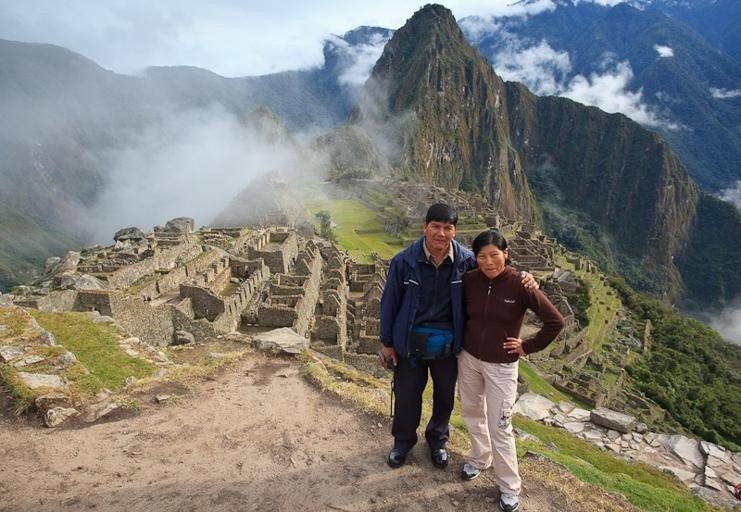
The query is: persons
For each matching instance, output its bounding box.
[457,230,566,511]
[379,201,541,466]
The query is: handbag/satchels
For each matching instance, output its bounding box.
[411,327,456,361]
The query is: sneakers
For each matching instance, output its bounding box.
[462,463,481,480]
[500,492,520,511]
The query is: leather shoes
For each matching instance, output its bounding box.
[388,441,418,468]
[431,444,449,469]
